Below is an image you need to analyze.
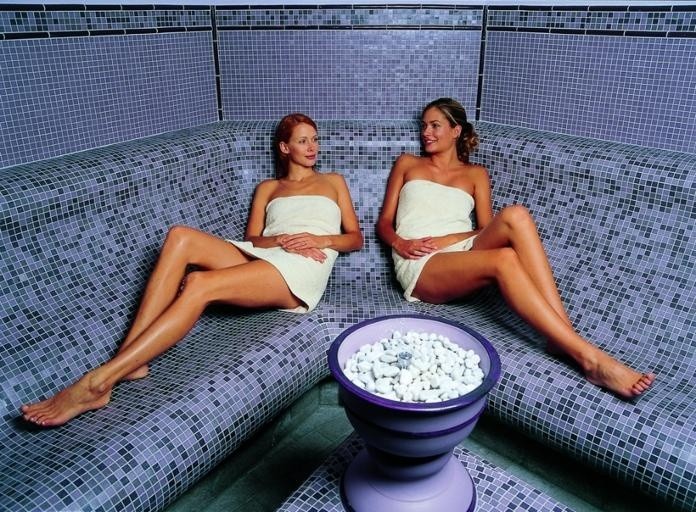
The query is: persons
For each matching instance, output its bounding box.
[373,96,655,399]
[18,112,365,429]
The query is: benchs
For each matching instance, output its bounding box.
[0,120,695,512]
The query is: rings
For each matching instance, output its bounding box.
[302,240,307,246]
